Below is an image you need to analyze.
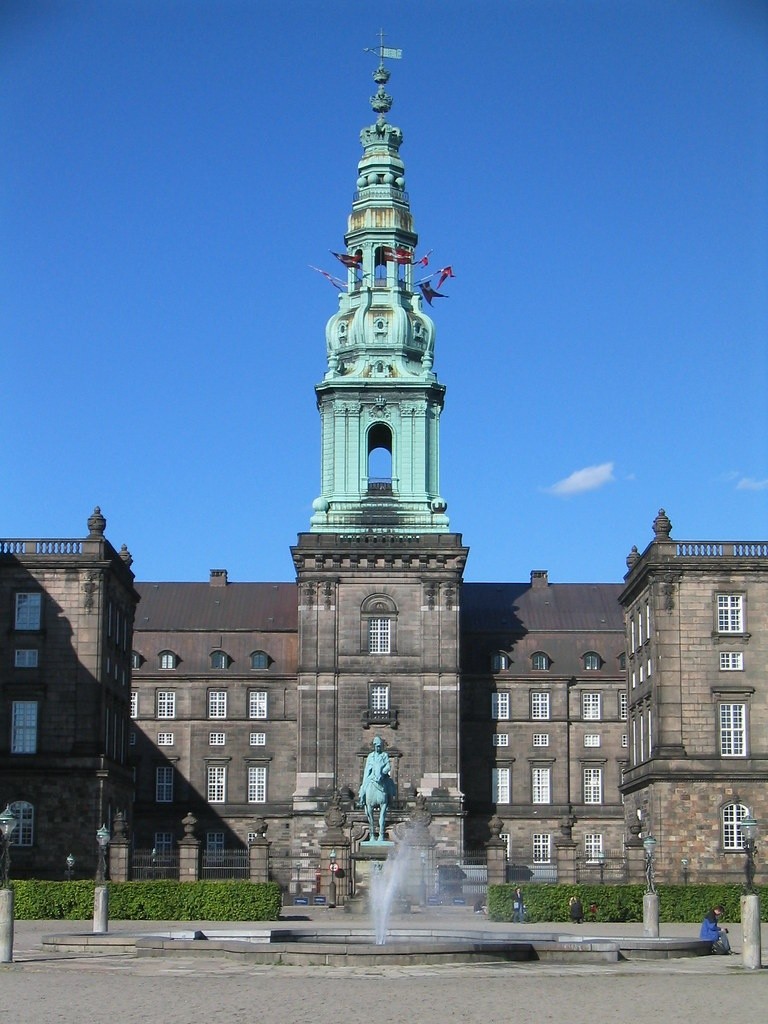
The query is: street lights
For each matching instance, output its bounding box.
[92,823,111,932]
[740,815,761,969]
[419,849,427,907]
[643,836,660,938]
[0,802,19,962]
[681,857,689,884]
[66,852,75,881]
[151,847,157,879]
[295,861,301,893]
[597,853,606,885]
[329,848,336,908]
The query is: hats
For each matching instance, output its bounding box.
[514,885,523,892]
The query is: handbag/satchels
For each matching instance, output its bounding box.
[523,909,527,913]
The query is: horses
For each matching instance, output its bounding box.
[363,758,396,842]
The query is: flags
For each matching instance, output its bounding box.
[319,248,454,308]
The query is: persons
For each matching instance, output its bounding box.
[474,900,485,914]
[568,895,584,922]
[356,737,396,811]
[512,887,528,923]
[701,906,736,954]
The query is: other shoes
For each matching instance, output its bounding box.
[520,921,525,923]
[726,950,735,955]
[573,921,581,924]
[513,921,518,923]
